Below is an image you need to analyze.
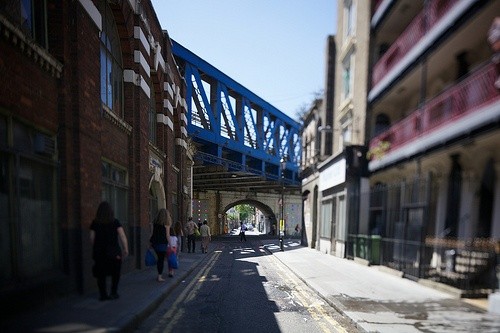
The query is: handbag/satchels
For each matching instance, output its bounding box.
[145,249,156,265]
[168,250,179,268]
[195,229,200,237]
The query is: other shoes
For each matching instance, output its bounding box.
[169,274,173,277]
[110,293,119,297]
[99,296,110,301]
[205,252,207,254]
[202,248,204,254]
[158,277,164,281]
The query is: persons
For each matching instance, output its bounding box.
[295,224,300,232]
[184,216,199,254]
[200,220,211,254]
[223,222,229,240]
[89,201,128,302]
[239,221,247,242]
[168,227,179,278]
[268,222,277,236]
[174,220,184,252]
[149,207,171,282]
[197,219,202,232]
